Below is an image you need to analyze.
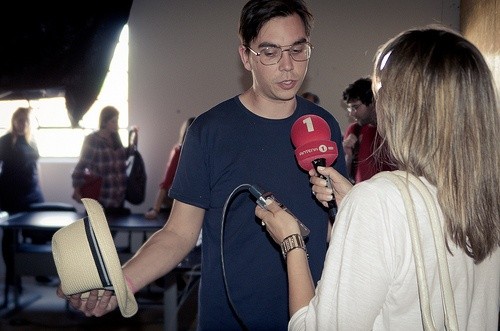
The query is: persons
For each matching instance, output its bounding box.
[255,27,500,331]
[0,0,384,331]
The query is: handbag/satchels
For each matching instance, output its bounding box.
[124,150,146,204]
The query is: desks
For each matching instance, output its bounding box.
[0,211,200,331]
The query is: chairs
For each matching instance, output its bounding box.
[3,203,77,311]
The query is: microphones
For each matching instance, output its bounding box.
[290,114,338,226]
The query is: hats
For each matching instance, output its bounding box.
[51,198,139,319]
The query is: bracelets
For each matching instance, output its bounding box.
[281,234,309,260]
[149,207,157,212]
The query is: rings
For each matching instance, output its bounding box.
[261,219,265,226]
[312,191,316,195]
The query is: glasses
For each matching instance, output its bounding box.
[244,41,315,66]
